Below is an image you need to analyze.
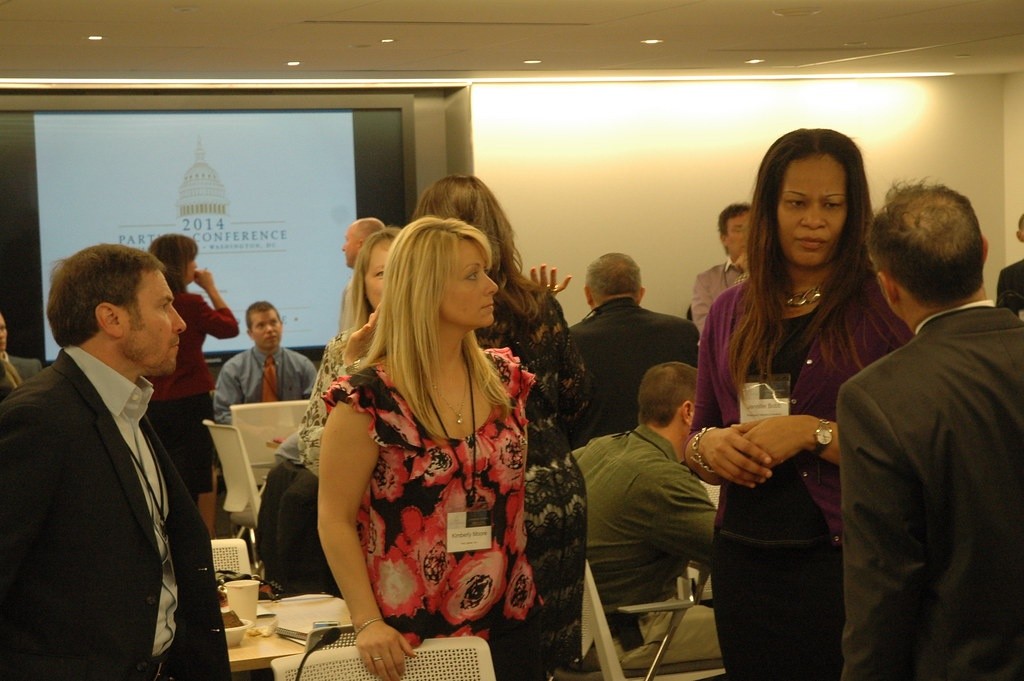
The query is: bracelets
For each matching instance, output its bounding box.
[689,427,720,473]
[354,618,384,638]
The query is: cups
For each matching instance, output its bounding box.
[224,580,260,622]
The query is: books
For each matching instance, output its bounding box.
[274,625,313,640]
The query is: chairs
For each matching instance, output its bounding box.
[582,560,694,681]
[211,538,252,581]
[271,634,496,681]
[202,419,267,539]
[230,401,311,540]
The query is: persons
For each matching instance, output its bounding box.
[144,234,317,510]
[837,175,1024,681]
[256,173,755,681]
[0,244,233,681]
[996,212,1024,321]
[684,128,916,681]
[0,313,43,402]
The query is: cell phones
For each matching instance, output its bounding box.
[313,621,339,630]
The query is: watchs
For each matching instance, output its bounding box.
[810,419,834,456]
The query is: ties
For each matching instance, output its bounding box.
[263,357,278,402]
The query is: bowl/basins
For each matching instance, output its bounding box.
[224,619,255,646]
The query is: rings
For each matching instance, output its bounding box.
[373,657,382,662]
[547,284,558,293]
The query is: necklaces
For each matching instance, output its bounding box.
[432,363,467,424]
[786,284,825,306]
[250,347,285,401]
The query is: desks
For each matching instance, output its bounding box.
[219,593,352,673]
